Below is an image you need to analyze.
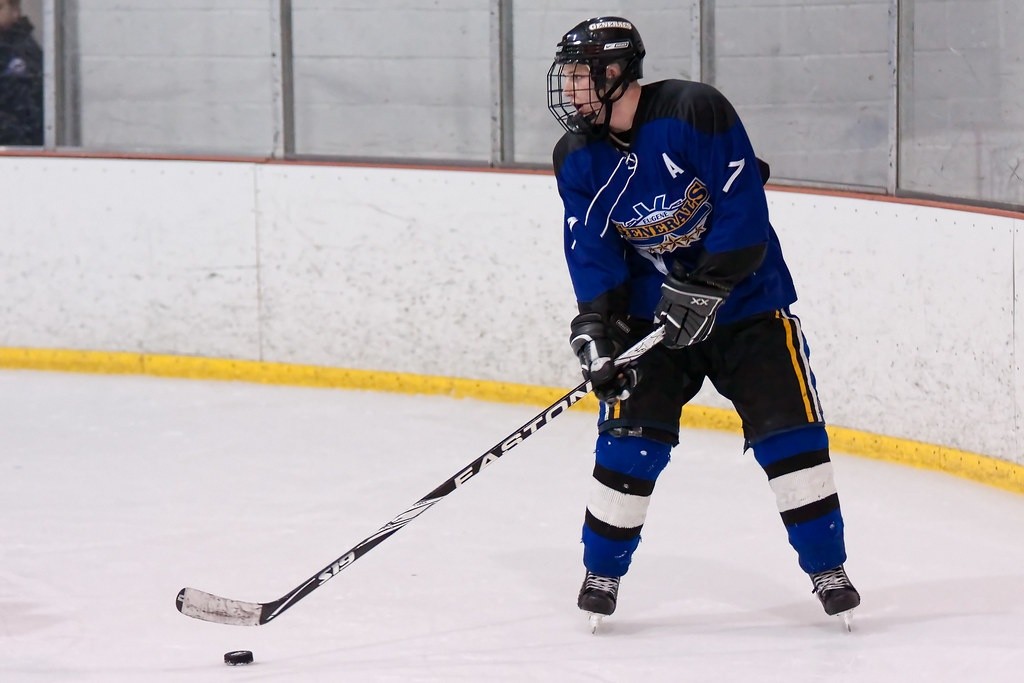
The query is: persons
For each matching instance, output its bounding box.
[547,16,860,637]
[0,0,45,146]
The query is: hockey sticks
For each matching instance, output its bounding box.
[173,324,662,628]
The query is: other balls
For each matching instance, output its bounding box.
[224,652,253,668]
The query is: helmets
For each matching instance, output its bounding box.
[555,16,646,87]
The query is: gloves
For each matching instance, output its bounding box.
[570,313,637,408]
[653,260,733,349]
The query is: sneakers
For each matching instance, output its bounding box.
[809,564,860,632]
[578,570,620,634]
